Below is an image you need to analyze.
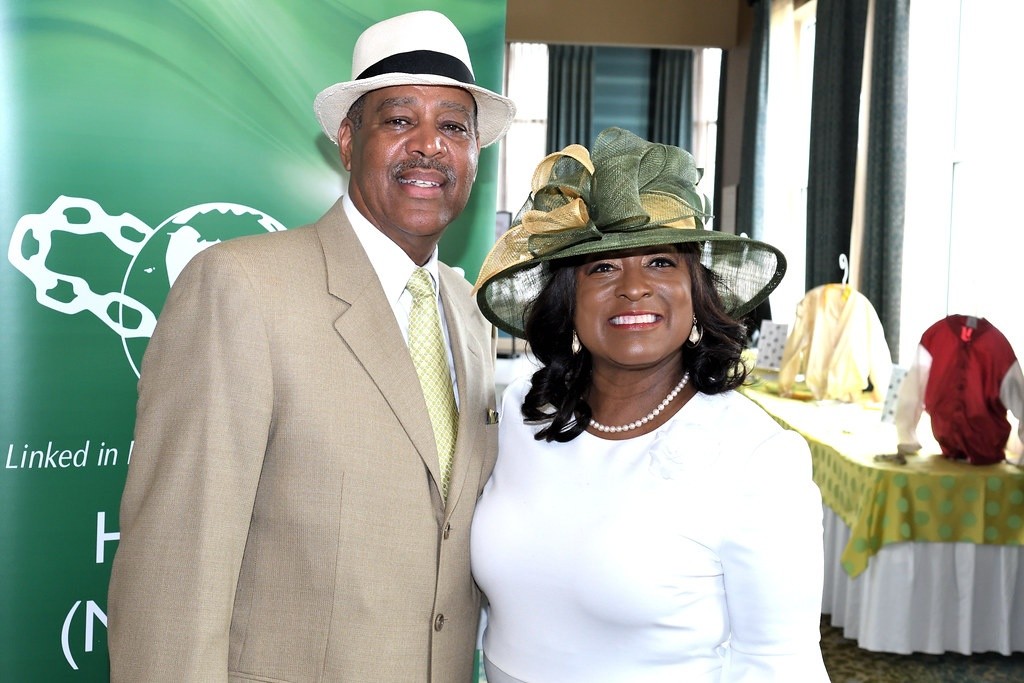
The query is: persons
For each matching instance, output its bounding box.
[106,10,518,683]
[469,130,832,683]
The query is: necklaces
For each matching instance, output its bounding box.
[587,372,689,433]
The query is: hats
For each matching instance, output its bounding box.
[468,128,788,340]
[315,11,516,148]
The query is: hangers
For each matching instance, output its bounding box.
[838,253,850,288]
[738,231,750,262]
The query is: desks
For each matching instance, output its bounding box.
[729,350,1024,657]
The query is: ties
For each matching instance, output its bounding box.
[404,268,460,510]
